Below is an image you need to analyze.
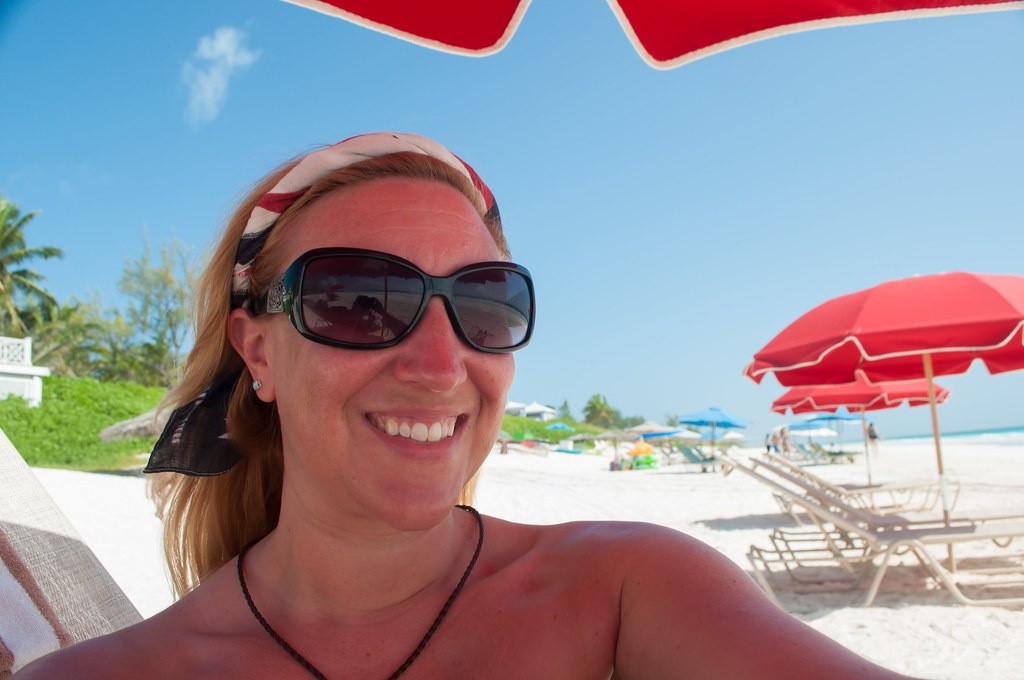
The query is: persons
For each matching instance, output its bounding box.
[0,121,930,679]
[867,422,879,457]
[760,423,792,464]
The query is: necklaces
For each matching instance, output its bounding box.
[235,502,492,679]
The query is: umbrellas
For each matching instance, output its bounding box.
[627,404,747,476]
[770,373,948,513]
[544,421,577,433]
[787,405,872,472]
[741,269,1024,580]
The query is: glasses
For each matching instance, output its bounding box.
[245,245,537,353]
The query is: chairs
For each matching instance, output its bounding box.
[798,444,827,461]
[748,454,1024,584]
[660,447,685,466]
[715,451,1024,615]
[766,447,962,514]
[692,444,720,460]
[674,443,719,474]
[815,441,863,463]
